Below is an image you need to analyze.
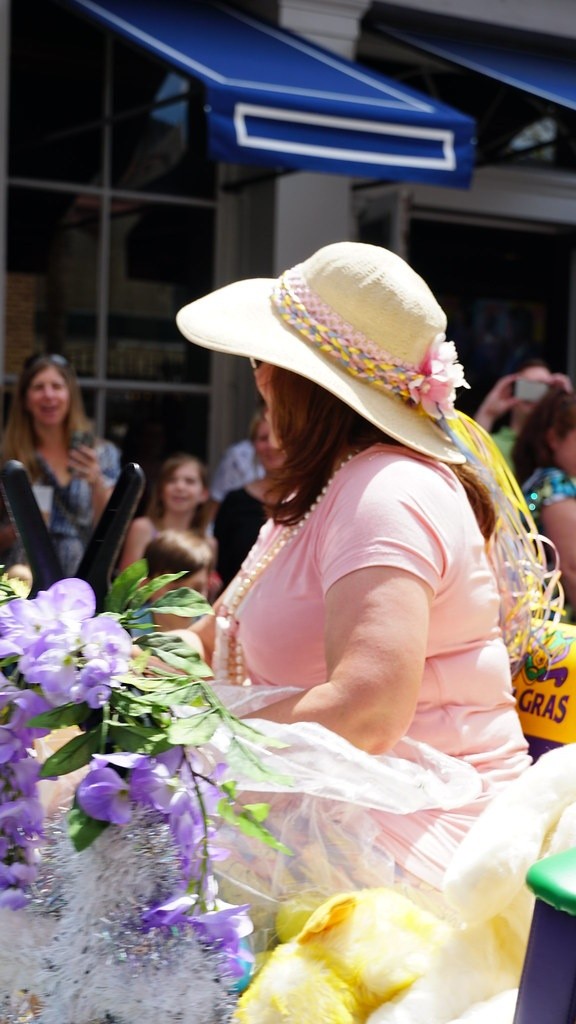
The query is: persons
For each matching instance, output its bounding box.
[470,361,576,626]
[121,238,540,948]
[0,350,120,602]
[117,451,225,609]
[197,394,285,607]
[123,530,218,642]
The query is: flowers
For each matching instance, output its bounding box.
[409,331,471,420]
[0,559,295,1024]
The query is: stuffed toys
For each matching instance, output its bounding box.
[222,732,576,1024]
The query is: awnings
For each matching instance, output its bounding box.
[379,22,576,110]
[75,0,476,190]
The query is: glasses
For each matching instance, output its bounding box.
[23,351,70,370]
[248,358,262,370]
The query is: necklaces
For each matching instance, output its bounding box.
[215,446,365,689]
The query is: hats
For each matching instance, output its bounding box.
[175,240,569,683]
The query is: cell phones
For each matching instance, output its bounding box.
[514,376,550,401]
[66,429,94,478]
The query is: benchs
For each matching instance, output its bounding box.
[454,617,576,1024]
